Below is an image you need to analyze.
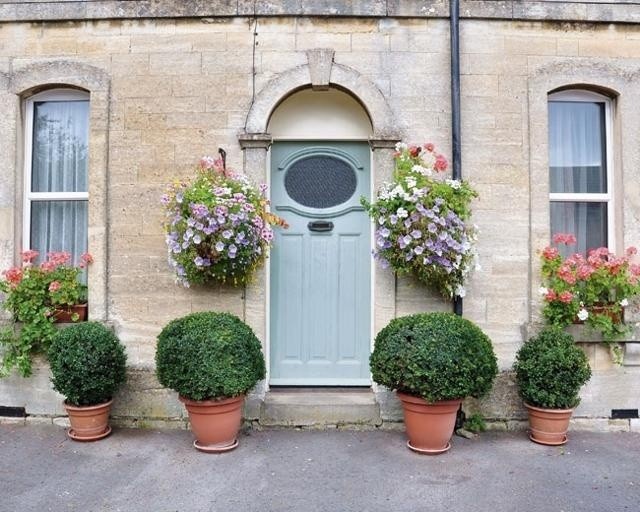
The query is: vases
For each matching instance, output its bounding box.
[42,302,86,322]
[570,301,624,326]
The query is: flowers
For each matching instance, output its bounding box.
[359,141,495,303]
[0,248,94,374]
[538,233,640,366]
[160,144,290,301]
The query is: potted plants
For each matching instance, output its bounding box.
[370,315,591,455]
[46,311,267,453]
